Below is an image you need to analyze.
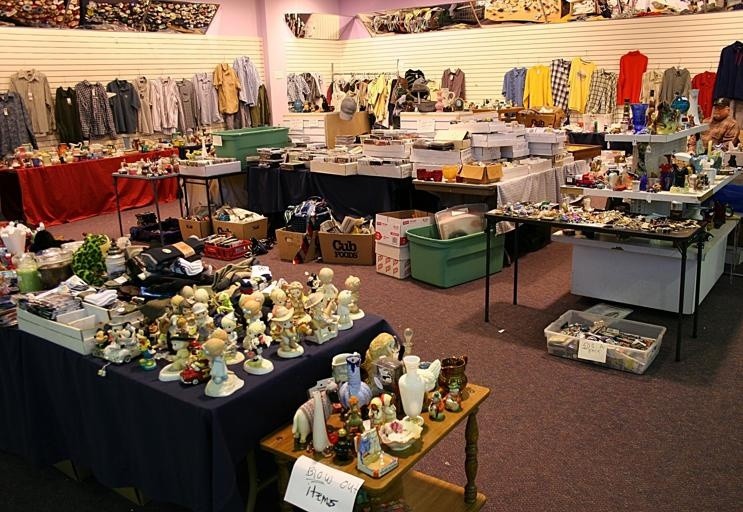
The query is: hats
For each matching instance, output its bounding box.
[339,98,356,121]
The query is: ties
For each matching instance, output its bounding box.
[292,215,312,265]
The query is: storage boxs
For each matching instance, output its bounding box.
[405,224,506,290]
[208,125,289,171]
[178,214,209,242]
[276,224,318,262]
[541,308,667,375]
[373,242,410,281]
[359,120,564,161]
[319,228,376,265]
[213,213,269,245]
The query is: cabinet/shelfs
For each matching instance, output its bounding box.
[554,121,742,316]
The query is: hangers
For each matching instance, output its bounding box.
[348,72,391,84]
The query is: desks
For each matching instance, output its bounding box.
[251,379,492,512]
[0,311,405,512]
[113,159,181,246]
[178,168,250,234]
[184,162,410,238]
[411,158,587,268]
[0,145,180,231]
[481,208,708,363]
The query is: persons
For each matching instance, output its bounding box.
[688,96,740,152]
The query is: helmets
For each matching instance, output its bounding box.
[712,97,730,107]
